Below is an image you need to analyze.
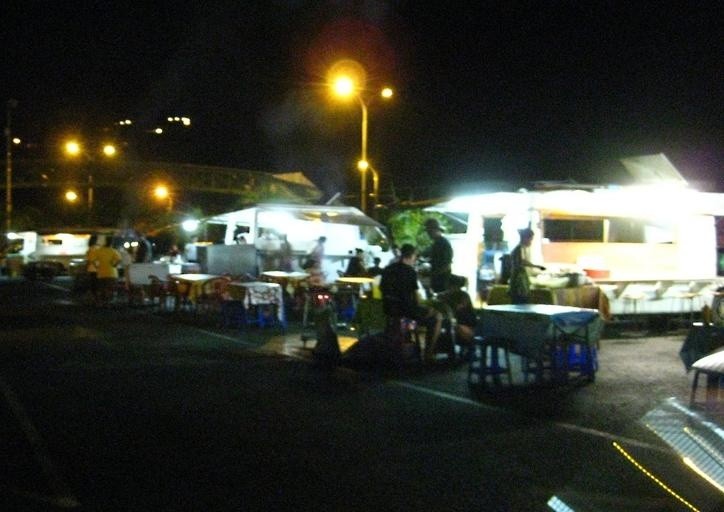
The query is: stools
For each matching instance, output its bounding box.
[383,281,610,387]
[19,255,375,335]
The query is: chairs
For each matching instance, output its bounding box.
[690,350,722,414]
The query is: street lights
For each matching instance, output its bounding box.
[63,136,117,230]
[355,158,380,218]
[324,65,395,217]
[153,184,173,218]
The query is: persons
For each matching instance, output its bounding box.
[184,234,200,263]
[346,247,366,297]
[91,236,121,309]
[378,244,444,364]
[113,237,131,305]
[308,236,326,263]
[370,257,383,278]
[304,260,323,286]
[436,274,476,360]
[83,234,98,307]
[505,227,547,303]
[422,218,454,300]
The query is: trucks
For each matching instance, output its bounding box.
[29,233,91,261]
[424,190,724,328]
[202,205,361,255]
